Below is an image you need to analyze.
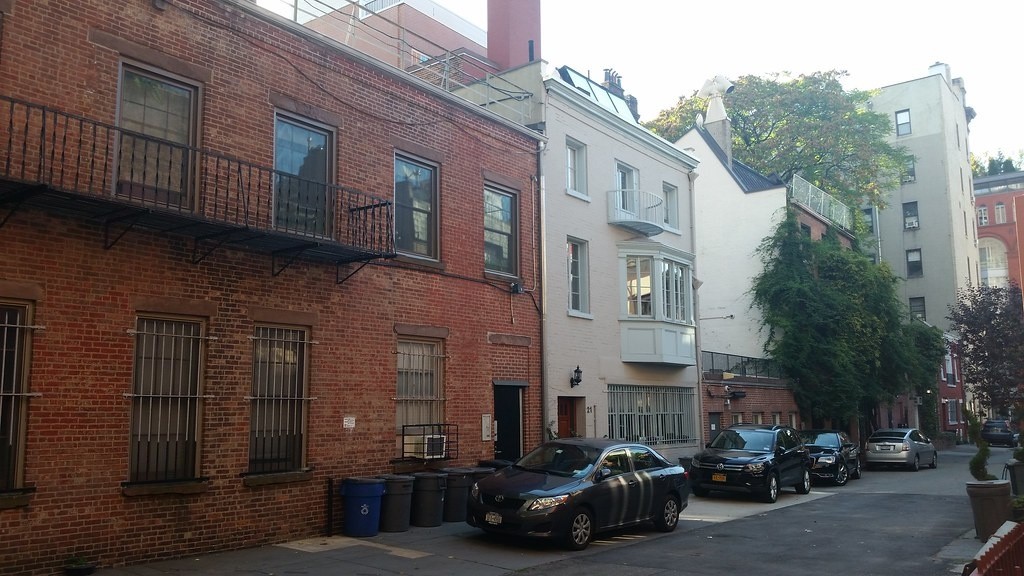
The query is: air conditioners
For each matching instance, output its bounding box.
[404,435,446,459]
[903,176,911,181]
[906,221,918,229]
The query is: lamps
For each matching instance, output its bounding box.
[571,365,583,388]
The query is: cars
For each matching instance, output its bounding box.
[864,427,937,472]
[465,437,689,552]
[800,428,862,486]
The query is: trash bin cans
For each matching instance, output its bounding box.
[440,467,473,522]
[340,475,384,537]
[413,470,450,527]
[965,479,1012,542]
[1004,459,1024,495]
[375,474,415,533]
[678,456,692,470]
[472,458,511,482]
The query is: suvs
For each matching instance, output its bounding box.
[689,424,812,504]
[981,419,1015,448]
